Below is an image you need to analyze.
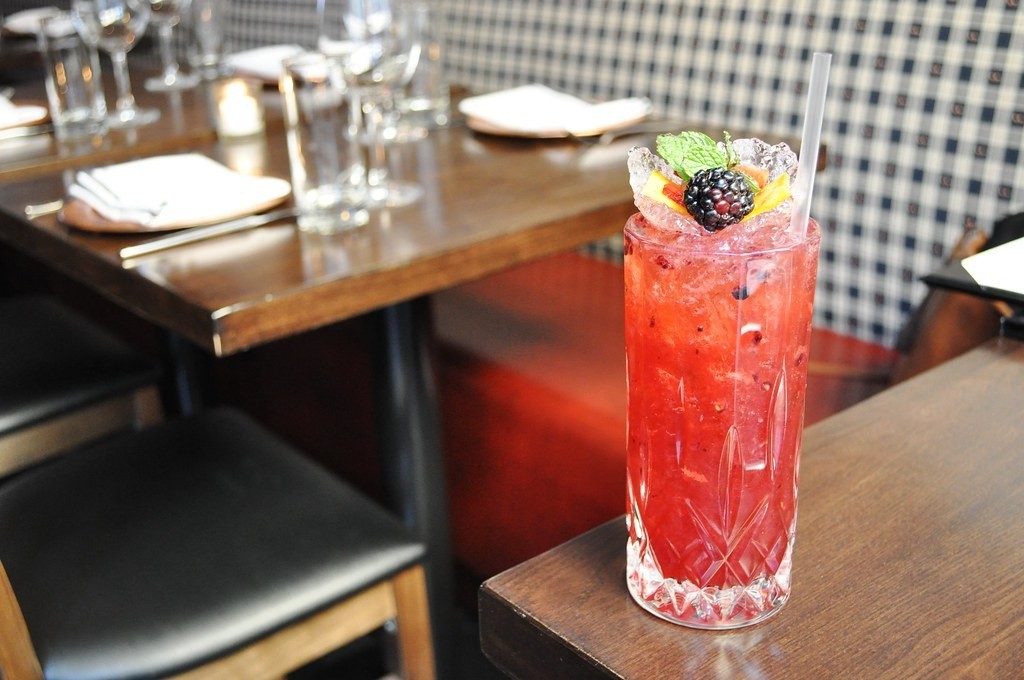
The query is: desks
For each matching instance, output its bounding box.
[478,332,1023,680]
[1,69,734,677]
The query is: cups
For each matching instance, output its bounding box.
[623,212,821,631]
[39,10,109,144]
[207,78,266,142]
[180,0,232,82]
[277,0,452,235]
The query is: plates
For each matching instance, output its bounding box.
[0,100,50,131]
[57,174,292,233]
[467,115,647,138]
[230,67,302,85]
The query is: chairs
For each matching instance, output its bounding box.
[1,242,438,680]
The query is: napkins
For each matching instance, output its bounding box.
[457,83,648,136]
[69,152,261,226]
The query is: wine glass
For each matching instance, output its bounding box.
[143,0,201,93]
[72,0,161,128]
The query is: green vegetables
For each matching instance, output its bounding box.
[655,127,760,195]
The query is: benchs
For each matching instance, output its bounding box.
[126,0,1024,582]
